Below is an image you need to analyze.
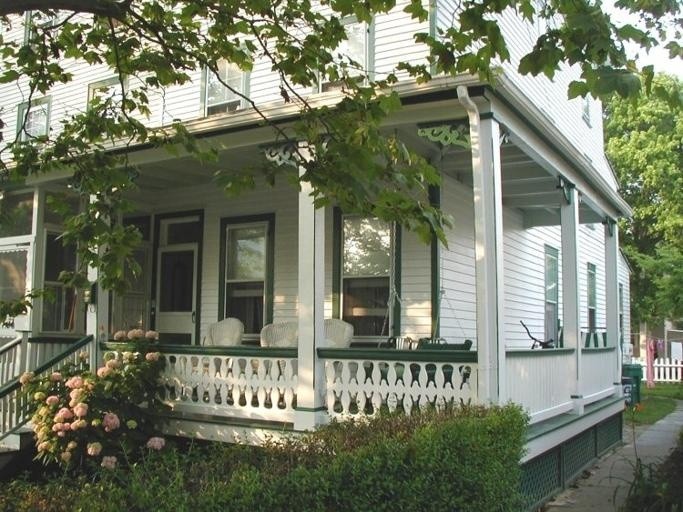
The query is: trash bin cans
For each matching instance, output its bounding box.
[621,364,643,408]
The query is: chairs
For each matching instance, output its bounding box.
[203,315,244,349]
[364,337,472,385]
[324,318,354,349]
[259,319,298,349]
